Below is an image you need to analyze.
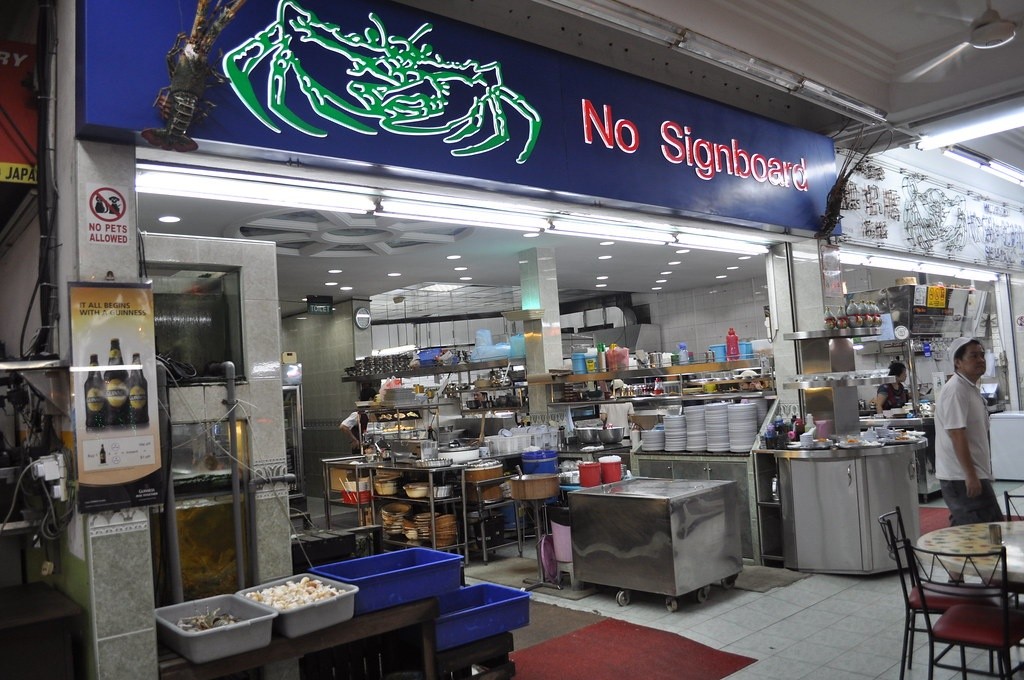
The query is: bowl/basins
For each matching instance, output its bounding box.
[377,482,451,498]
[498,425,556,437]
[787,369,890,381]
[559,471,580,484]
[381,504,456,546]
[575,427,624,444]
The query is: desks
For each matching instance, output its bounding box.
[915,520,1024,646]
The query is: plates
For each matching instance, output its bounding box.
[641,402,757,452]
[467,400,480,409]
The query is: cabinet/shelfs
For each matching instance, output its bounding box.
[457,475,522,562]
[320,457,371,530]
[357,462,468,564]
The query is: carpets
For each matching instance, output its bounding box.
[509,614,757,680]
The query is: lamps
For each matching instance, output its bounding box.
[136,0,1024,285]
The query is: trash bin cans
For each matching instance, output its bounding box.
[549,506,573,562]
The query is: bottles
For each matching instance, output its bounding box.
[100,445,105,463]
[703,375,772,393]
[486,393,519,408]
[822,300,883,329]
[914,338,952,359]
[104,339,130,426]
[129,353,149,424]
[727,328,740,361]
[858,399,876,410]
[413,384,438,399]
[765,414,814,449]
[85,355,105,427]
[630,423,641,451]
[622,378,688,397]
[490,367,509,388]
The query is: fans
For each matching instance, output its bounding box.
[894,0,1024,85]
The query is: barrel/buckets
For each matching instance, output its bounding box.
[601,462,622,484]
[522,450,559,504]
[571,353,588,375]
[579,462,601,486]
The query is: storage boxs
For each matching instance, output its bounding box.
[153,546,531,665]
[139,259,244,385]
[149,421,258,608]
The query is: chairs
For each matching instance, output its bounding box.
[878,490,1024,680]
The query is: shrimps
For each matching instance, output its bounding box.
[245,575,347,611]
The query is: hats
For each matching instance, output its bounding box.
[949,338,976,369]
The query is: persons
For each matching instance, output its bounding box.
[876,362,910,413]
[339,386,378,477]
[600,379,634,440]
[473,390,489,402]
[934,337,1004,524]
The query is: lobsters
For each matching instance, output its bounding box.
[179,600,238,634]
